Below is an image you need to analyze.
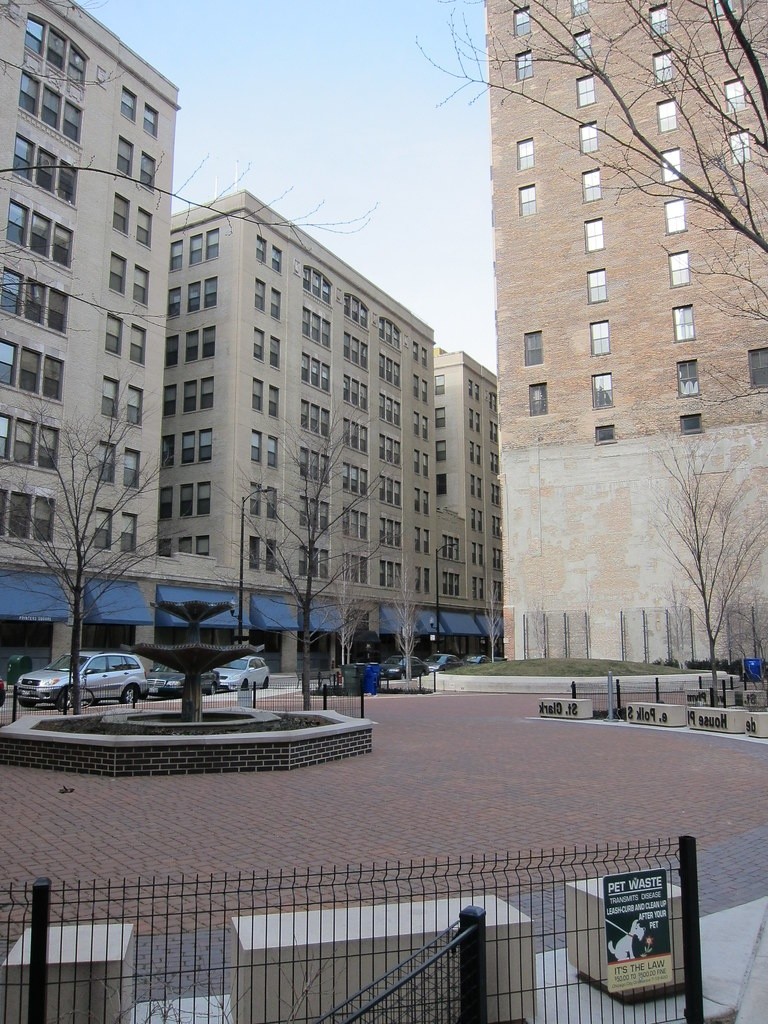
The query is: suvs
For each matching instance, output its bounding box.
[15,650,148,711]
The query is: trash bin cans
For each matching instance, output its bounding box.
[338,663,366,697]
[745,658,762,681]
[362,661,382,696]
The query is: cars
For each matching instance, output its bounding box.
[425,653,465,673]
[466,654,492,664]
[210,655,272,691]
[379,655,430,681]
[138,663,220,700]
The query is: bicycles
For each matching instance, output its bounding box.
[56,668,95,712]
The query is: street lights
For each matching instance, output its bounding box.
[230,488,275,645]
[428,542,458,653]
